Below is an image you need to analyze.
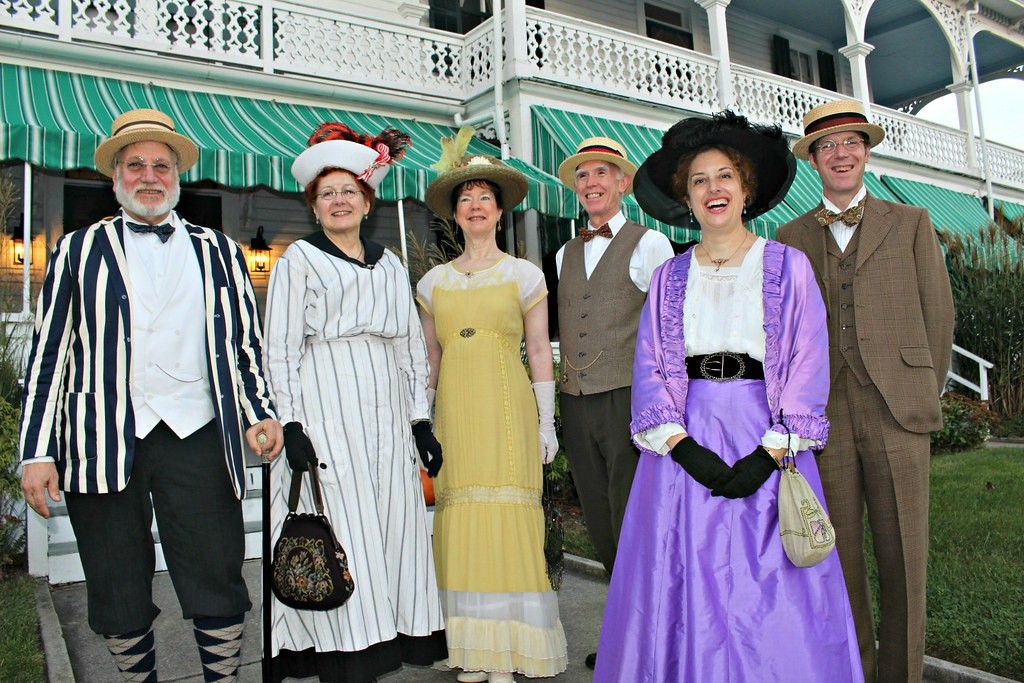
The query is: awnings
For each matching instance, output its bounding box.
[0,62,580,219]
[880,175,1024,272]
[531,104,946,259]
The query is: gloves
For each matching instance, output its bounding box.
[411,421,443,479]
[283,421,319,472]
[670,436,736,490]
[710,444,780,499]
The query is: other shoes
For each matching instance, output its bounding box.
[318,675,376,683]
[458,670,488,683]
[488,672,514,683]
[585,652,597,668]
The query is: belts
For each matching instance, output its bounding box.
[686,352,764,380]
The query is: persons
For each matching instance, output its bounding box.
[416,155,560,683]
[776,100,955,683]
[20,109,284,683]
[555,136,676,670]
[262,139,443,683]
[630,110,831,682]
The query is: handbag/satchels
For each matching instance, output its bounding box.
[543,454,565,592]
[270,459,355,611]
[777,421,836,568]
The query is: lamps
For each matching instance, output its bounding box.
[248,226,272,272]
[10,212,35,264]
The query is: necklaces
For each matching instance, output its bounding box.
[356,233,365,262]
[701,231,747,272]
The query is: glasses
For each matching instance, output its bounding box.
[116,156,176,173]
[811,136,866,151]
[314,188,364,200]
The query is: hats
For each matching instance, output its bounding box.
[558,136,639,198]
[792,101,886,160]
[632,110,797,230]
[94,109,198,179]
[424,125,529,222]
[291,121,413,192]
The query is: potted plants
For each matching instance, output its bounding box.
[524,360,567,593]
[389,214,530,505]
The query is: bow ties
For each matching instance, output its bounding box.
[817,206,863,226]
[125,221,176,243]
[579,222,614,242]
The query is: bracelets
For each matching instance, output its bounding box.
[411,419,433,431]
[762,446,782,470]
